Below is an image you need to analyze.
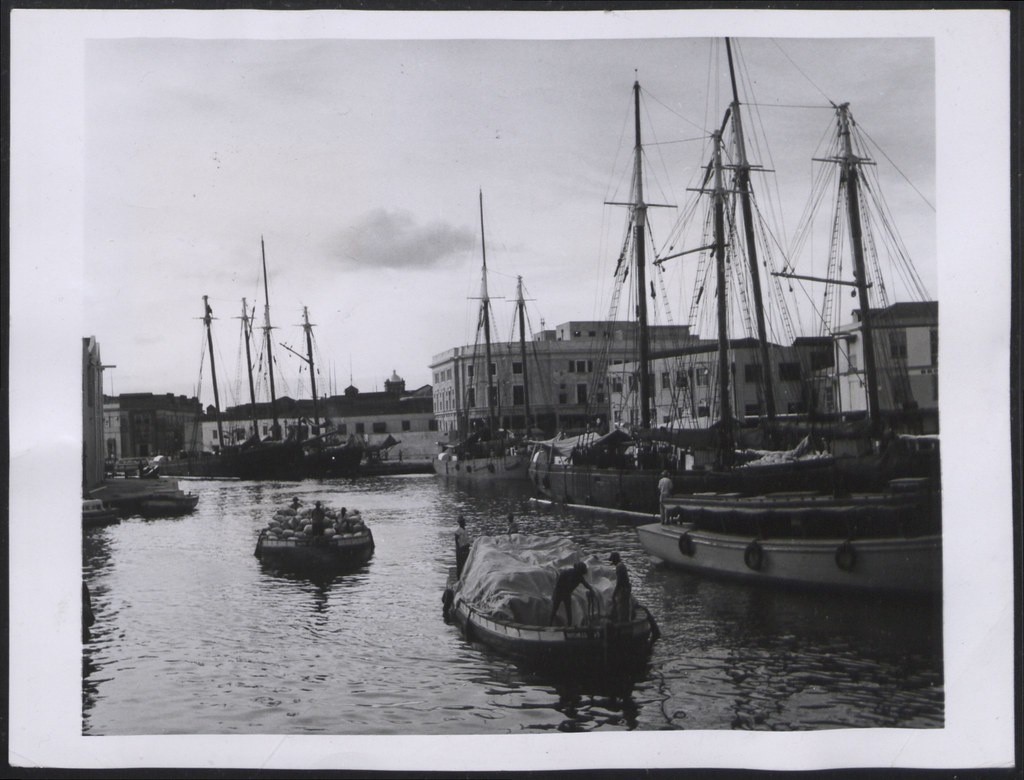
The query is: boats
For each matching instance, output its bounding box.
[105,475,201,520]
[256,511,375,586]
[442,534,660,662]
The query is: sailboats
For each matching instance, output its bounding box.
[431,190,556,477]
[147,233,402,482]
[633,37,939,586]
[528,63,727,512]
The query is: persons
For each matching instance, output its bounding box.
[291,498,362,537]
[454,516,470,580]
[608,553,631,626]
[657,470,673,524]
[506,514,518,536]
[549,562,594,627]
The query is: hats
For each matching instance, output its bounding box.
[608,552,622,562]
[574,562,588,574]
[661,470,670,477]
[293,497,299,501]
[315,501,322,505]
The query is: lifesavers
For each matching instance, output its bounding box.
[743,543,764,570]
[678,534,694,556]
[835,542,858,570]
